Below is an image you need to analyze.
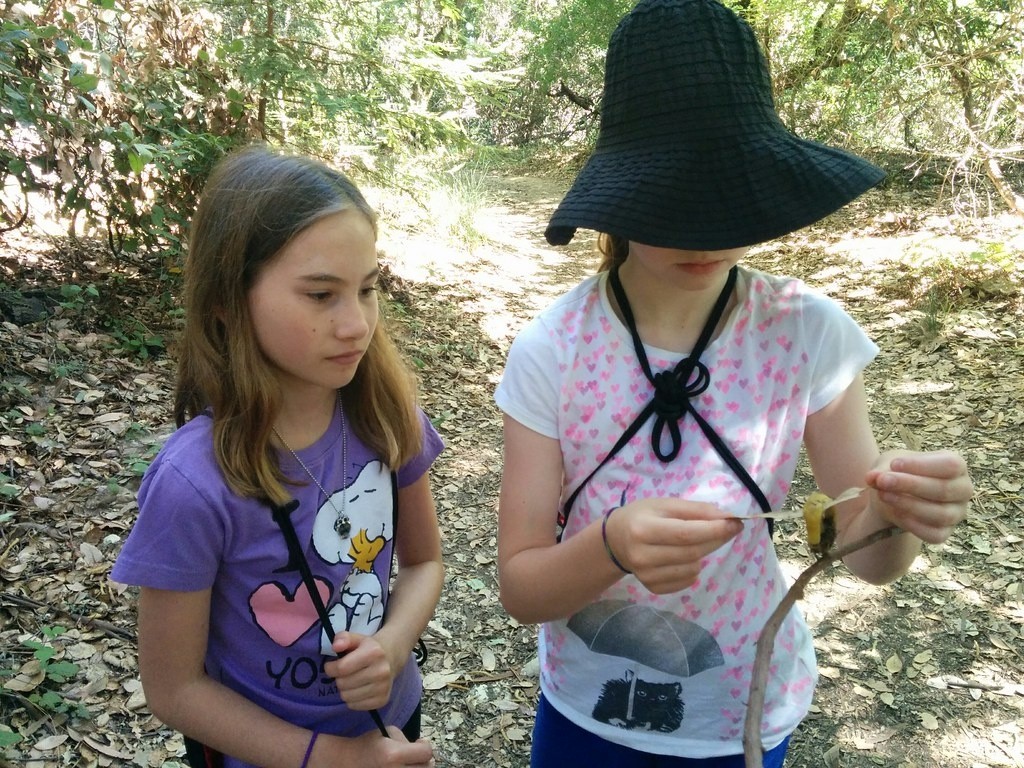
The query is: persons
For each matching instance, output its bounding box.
[493,0,975,768]
[111,142,446,768]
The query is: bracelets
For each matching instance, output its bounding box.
[301,729,318,768]
[603,507,632,575]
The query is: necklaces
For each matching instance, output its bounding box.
[271,390,352,539]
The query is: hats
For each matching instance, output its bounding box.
[544,0,888,252]
